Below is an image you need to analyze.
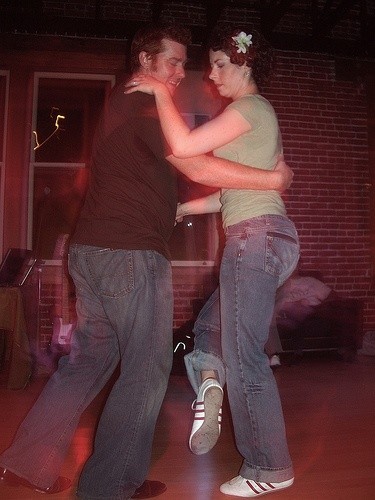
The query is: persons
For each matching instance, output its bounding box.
[124,25,300,497]
[0,20,294,500]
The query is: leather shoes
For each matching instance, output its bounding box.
[0,467,71,494]
[128,481,166,499]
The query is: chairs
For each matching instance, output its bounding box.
[0,249,42,390]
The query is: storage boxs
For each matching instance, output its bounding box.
[270,298,365,362]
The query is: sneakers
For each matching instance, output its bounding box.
[220,474,295,497]
[189,378,224,455]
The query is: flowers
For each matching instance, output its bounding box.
[232,31,254,54]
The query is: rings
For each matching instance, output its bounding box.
[134,81,136,85]
